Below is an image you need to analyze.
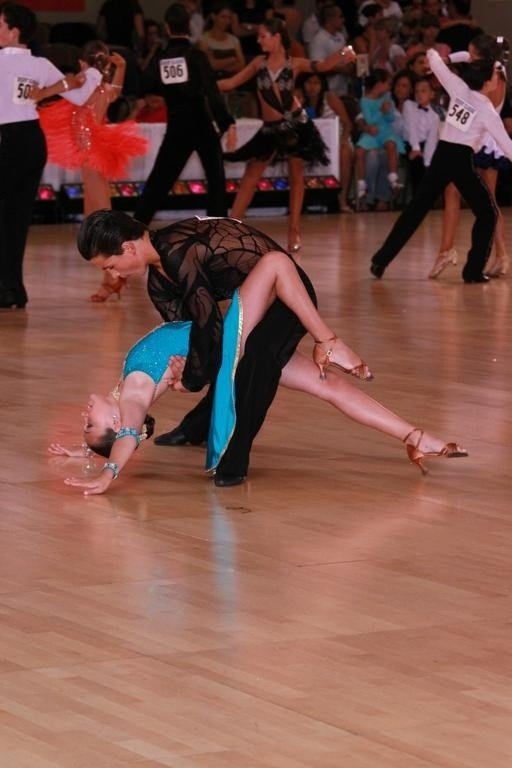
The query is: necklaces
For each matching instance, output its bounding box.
[109,383,121,402]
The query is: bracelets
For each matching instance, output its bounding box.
[80,443,95,457]
[101,463,120,482]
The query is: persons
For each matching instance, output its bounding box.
[131,1,512,282]
[1,0,151,311]
[45,251,471,501]
[76,206,320,488]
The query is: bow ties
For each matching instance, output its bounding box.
[417,104,428,112]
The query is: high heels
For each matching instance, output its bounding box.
[428,249,458,278]
[87,281,122,303]
[287,227,303,252]
[312,335,374,381]
[403,428,469,475]
[486,256,509,275]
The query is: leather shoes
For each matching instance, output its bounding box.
[0,290,27,309]
[462,271,491,283]
[371,255,385,278]
